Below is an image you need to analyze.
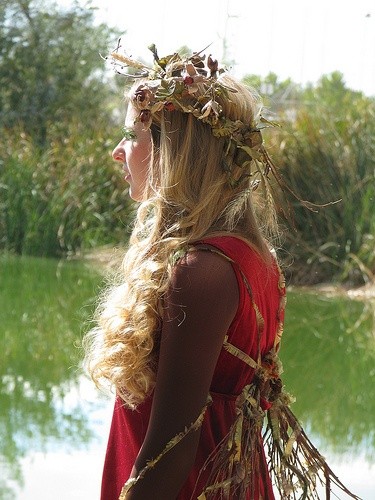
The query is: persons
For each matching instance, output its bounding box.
[78,37,291,500]
